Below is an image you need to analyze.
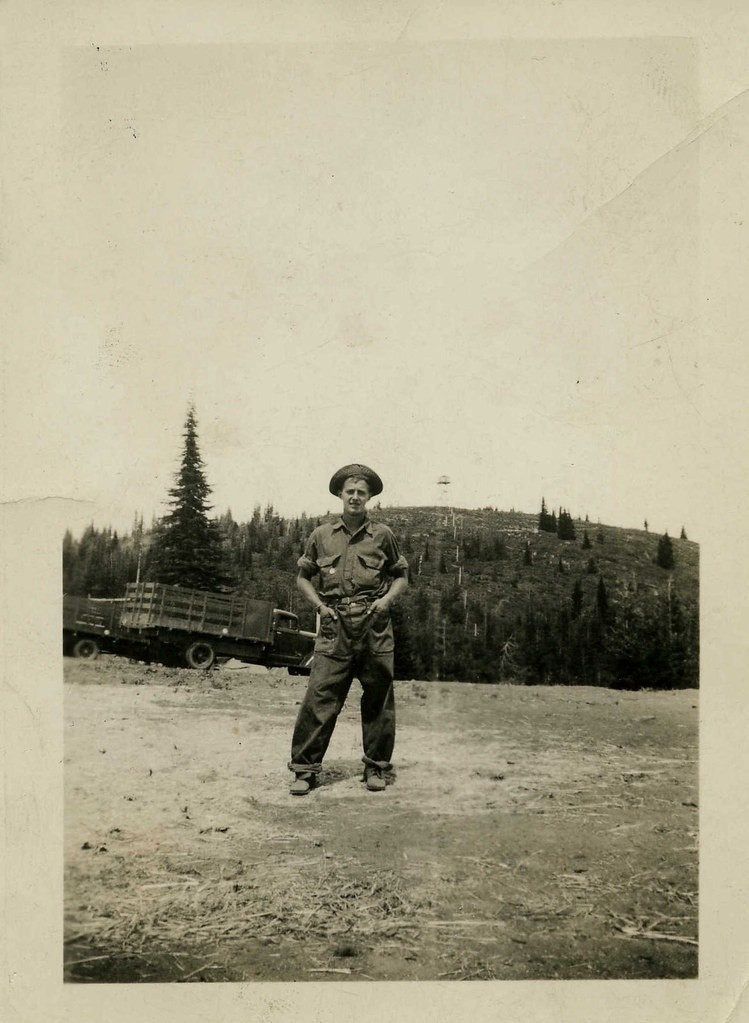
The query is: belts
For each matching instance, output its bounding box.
[332,594,375,604]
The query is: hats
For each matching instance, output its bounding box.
[328,463,383,500]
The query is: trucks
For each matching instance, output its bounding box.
[63,591,185,664]
[119,582,320,678]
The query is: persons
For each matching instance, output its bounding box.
[289,463,409,796]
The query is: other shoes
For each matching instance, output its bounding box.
[290,772,317,794]
[364,763,386,791]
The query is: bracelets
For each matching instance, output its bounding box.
[314,602,327,612]
